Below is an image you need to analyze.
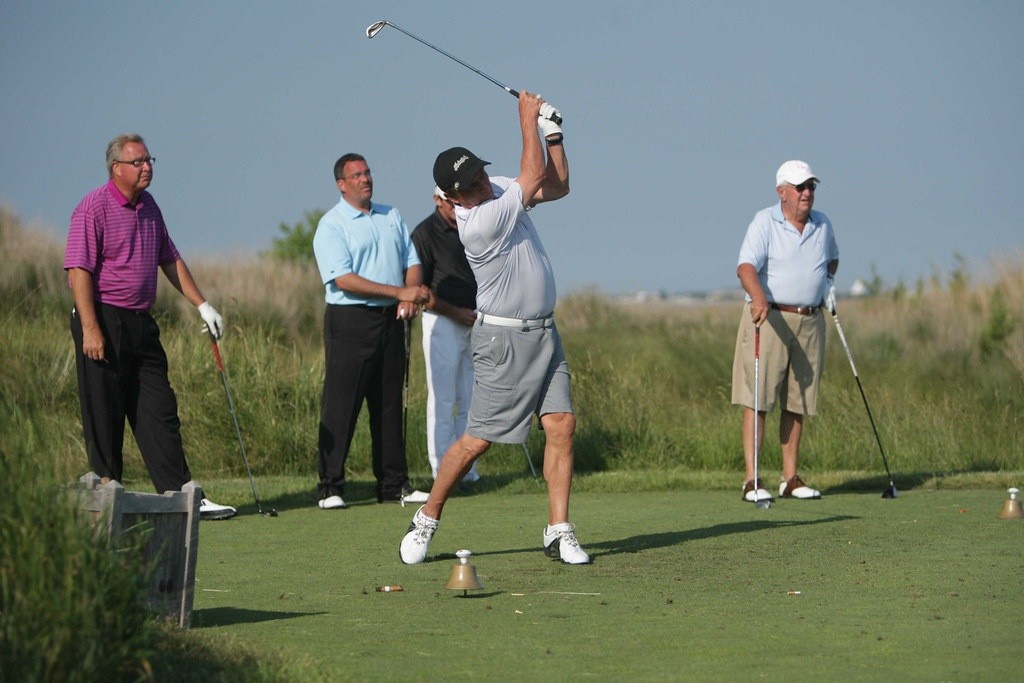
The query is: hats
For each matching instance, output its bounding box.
[433,146,492,193]
[775,160,820,186]
[435,184,448,201]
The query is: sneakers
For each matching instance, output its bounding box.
[779,475,821,499]
[399,504,440,565]
[742,478,773,502]
[543,523,589,564]
[318,489,345,509]
[379,483,430,503]
[199,498,237,520]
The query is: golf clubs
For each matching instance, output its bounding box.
[365,20,562,126]
[522,442,540,486]
[755,318,771,509]
[830,305,897,498]
[210,322,279,517]
[401,312,407,507]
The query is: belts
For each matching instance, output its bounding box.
[769,302,820,314]
[477,310,554,328]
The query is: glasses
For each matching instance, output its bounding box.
[117,158,155,167]
[786,183,817,192]
[344,170,374,180]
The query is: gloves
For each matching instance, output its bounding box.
[822,273,837,313]
[197,300,224,340]
[536,94,563,137]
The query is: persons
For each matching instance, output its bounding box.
[731,160,840,502]
[400,90,590,566]
[313,153,430,508]
[64,134,236,520]
[411,184,484,497]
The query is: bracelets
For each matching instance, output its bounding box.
[545,134,563,146]
